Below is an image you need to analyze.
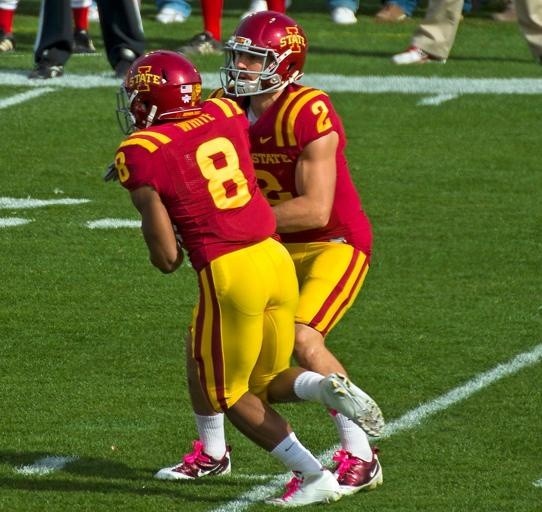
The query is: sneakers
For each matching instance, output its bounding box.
[112,55,131,78]
[241,1,271,20]
[73,31,95,52]
[154,379,385,507]
[377,4,407,20]
[28,64,65,81]
[333,8,357,23]
[154,5,187,24]
[174,33,223,56]
[0,37,16,52]
[393,48,446,65]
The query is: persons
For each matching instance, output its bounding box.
[105,48,386,507]
[0,0,145,80]
[156,2,292,53]
[150,8,387,501]
[331,0,542,67]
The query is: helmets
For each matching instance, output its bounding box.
[221,11,308,97]
[117,51,202,135]
